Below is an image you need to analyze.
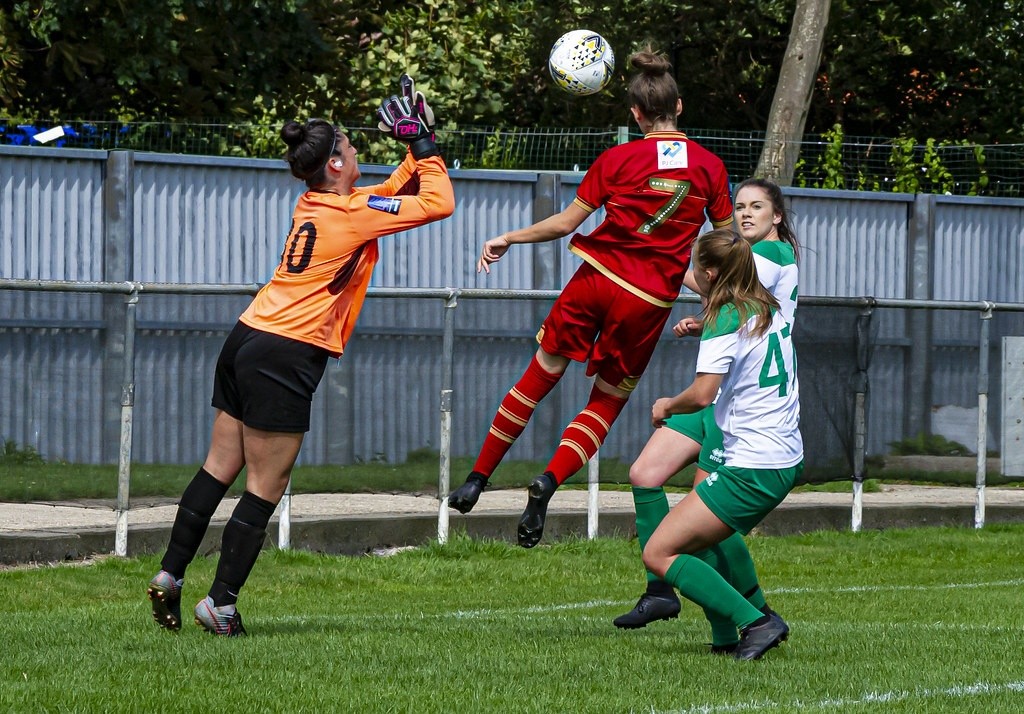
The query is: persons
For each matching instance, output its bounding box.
[448,41,733,550]
[612,178,801,629]
[148,74,456,642]
[643,229,807,663]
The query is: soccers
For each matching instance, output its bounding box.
[548,29,615,96]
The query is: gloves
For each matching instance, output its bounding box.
[376,90,438,160]
[401,74,436,143]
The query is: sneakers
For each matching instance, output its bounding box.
[517,470,558,549]
[735,614,790,660]
[194,592,248,637]
[763,609,784,622]
[447,471,489,515]
[613,592,681,629]
[146,569,185,631]
[708,640,740,655]
